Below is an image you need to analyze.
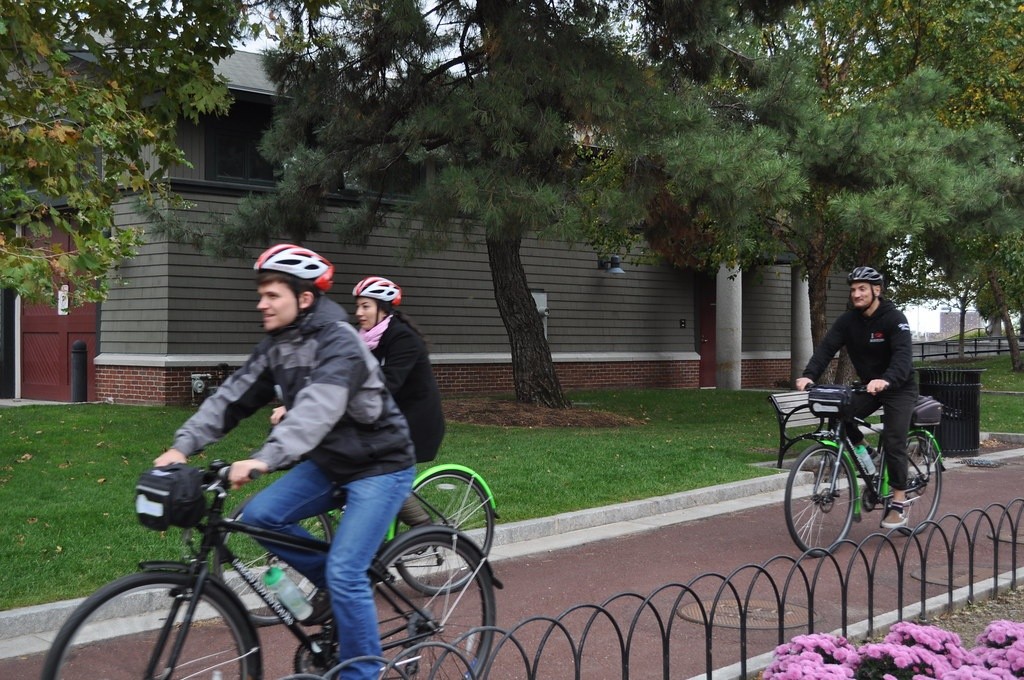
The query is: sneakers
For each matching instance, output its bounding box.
[881,500,910,529]
[300,588,333,627]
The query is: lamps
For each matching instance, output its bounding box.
[598,255,624,274]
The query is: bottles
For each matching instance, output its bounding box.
[262,567,313,621]
[854,445,876,477]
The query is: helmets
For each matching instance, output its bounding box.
[254,244,335,296]
[846,267,883,285]
[352,276,402,306]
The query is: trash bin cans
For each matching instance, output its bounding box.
[914,366,987,458]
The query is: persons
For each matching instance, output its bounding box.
[151,244,416,680]
[269,276,447,528]
[796,265,921,529]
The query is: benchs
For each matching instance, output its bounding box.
[767,389,885,468]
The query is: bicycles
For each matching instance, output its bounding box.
[784,380,946,556]
[43,459,503,680]
[213,425,500,628]
